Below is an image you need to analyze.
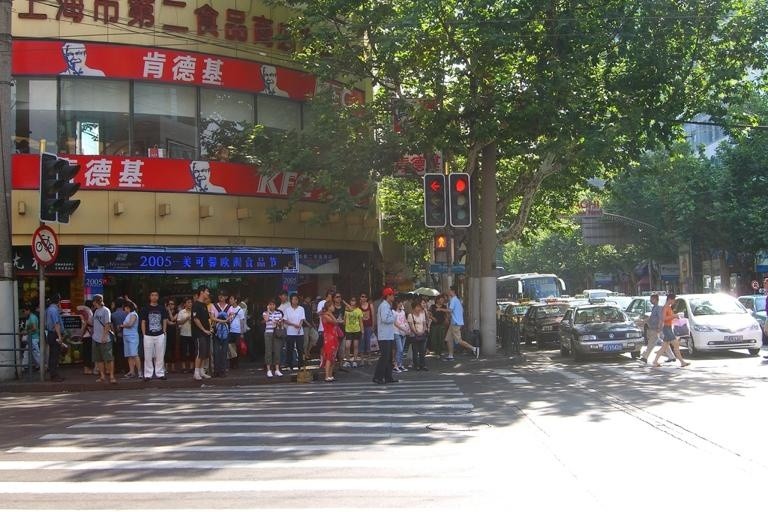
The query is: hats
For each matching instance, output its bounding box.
[93,293,105,306]
[383,288,398,297]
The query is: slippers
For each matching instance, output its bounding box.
[96,377,105,382]
[108,377,116,383]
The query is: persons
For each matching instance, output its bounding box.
[654,294,689,368]
[59,42,107,77]
[22,293,65,383]
[256,65,290,98]
[83,285,252,382]
[634,295,676,363]
[762,277,768,359]
[186,160,228,193]
[436,285,479,362]
[262,289,447,385]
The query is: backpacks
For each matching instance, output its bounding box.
[212,303,232,344]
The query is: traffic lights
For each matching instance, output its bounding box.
[434,233,449,263]
[38,151,66,223]
[423,173,447,228]
[449,173,471,228]
[57,157,81,225]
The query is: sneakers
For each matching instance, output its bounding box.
[193,372,202,380]
[343,361,351,368]
[352,361,358,368]
[138,373,143,379]
[124,372,135,378]
[200,371,211,379]
[392,363,428,373]
[274,370,283,376]
[266,370,273,377]
[442,357,455,361]
[472,346,479,359]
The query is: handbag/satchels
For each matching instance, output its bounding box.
[671,318,691,340]
[47,331,58,345]
[226,343,238,359]
[415,332,427,341]
[110,331,116,343]
[274,319,285,338]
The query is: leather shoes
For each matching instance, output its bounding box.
[51,375,63,382]
[385,377,399,382]
[218,371,224,378]
[639,358,692,368]
[372,377,385,384]
[213,372,218,378]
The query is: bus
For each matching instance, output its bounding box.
[496,272,566,301]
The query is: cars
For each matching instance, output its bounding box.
[667,293,762,357]
[737,296,767,345]
[625,296,667,348]
[559,304,643,360]
[522,302,573,351]
[496,289,675,322]
[499,304,530,341]
